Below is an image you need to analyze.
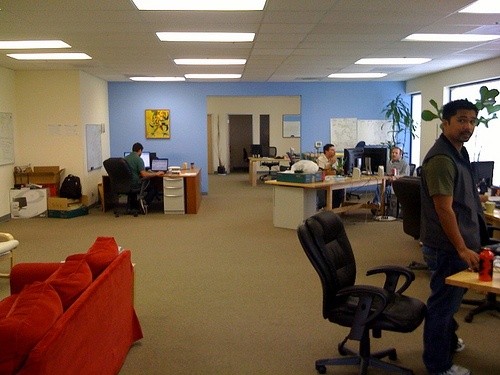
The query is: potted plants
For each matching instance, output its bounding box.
[380,93,419,197]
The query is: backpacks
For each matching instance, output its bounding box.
[59,174,81,199]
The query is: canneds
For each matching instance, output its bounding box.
[167,168,172,175]
[391,168,397,177]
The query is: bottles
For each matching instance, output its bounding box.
[479,247,494,281]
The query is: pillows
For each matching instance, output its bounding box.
[45,254,93,312]
[84,236,119,281]
[0,280,64,373]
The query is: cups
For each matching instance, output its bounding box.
[485,202,495,213]
[391,168,397,176]
[190,163,194,170]
[183,163,188,169]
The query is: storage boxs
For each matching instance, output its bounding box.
[28,165,66,196]
[15,163,32,187]
[47,195,88,219]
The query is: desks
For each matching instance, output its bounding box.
[101,168,202,215]
[482,207,500,224]
[264,173,402,230]
[445,256,500,295]
[248,157,301,187]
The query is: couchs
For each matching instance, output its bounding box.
[0,236,143,375]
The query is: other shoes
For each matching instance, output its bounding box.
[453,337,465,353]
[436,363,472,375]
[367,200,382,216]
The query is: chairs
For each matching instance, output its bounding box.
[102,157,150,218]
[371,163,416,219]
[296,211,427,375]
[392,177,500,324]
[242,144,281,182]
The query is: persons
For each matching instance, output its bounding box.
[419,100,489,375]
[316,144,338,175]
[119,142,164,215]
[368,148,410,215]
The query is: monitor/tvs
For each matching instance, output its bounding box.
[151,158,168,172]
[362,146,387,174]
[124,152,151,170]
[343,148,363,175]
[470,161,494,195]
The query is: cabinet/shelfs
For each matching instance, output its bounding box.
[163,177,185,215]
